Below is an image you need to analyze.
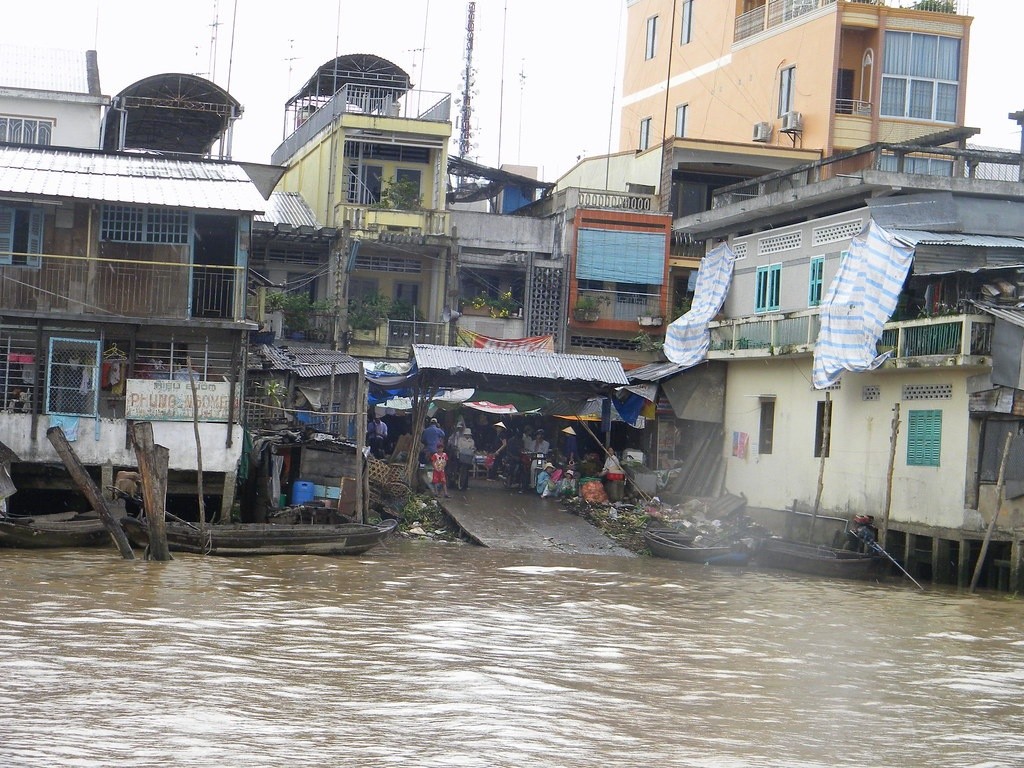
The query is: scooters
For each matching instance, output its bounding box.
[526,450,546,490]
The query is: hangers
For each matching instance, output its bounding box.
[104,352,127,362]
[66,350,83,364]
[149,357,165,370]
[103,343,125,355]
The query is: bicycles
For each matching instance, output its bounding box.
[446,445,475,491]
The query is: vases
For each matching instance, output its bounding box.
[461,305,500,316]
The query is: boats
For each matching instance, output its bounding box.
[0,502,129,547]
[644,527,754,565]
[755,535,895,579]
[118,511,399,557]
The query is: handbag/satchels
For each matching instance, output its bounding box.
[543,484,552,497]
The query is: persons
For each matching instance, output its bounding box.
[368,417,620,499]
[431,443,451,498]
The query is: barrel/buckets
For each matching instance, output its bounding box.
[293,480,314,505]
[338,477,357,516]
[281,494,287,507]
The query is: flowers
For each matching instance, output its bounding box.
[460,287,515,321]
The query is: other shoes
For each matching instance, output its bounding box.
[444,494,452,498]
[518,489,528,494]
[435,493,441,498]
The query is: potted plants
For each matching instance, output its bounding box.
[638,309,662,325]
[573,296,600,322]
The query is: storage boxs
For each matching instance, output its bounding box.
[337,477,357,517]
[606,473,625,481]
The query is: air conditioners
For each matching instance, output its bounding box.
[781,110,802,128]
[752,122,769,140]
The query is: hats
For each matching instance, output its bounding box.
[534,429,544,436]
[566,469,574,478]
[464,428,472,435]
[543,462,555,471]
[431,418,437,423]
[494,421,507,429]
[562,426,576,435]
